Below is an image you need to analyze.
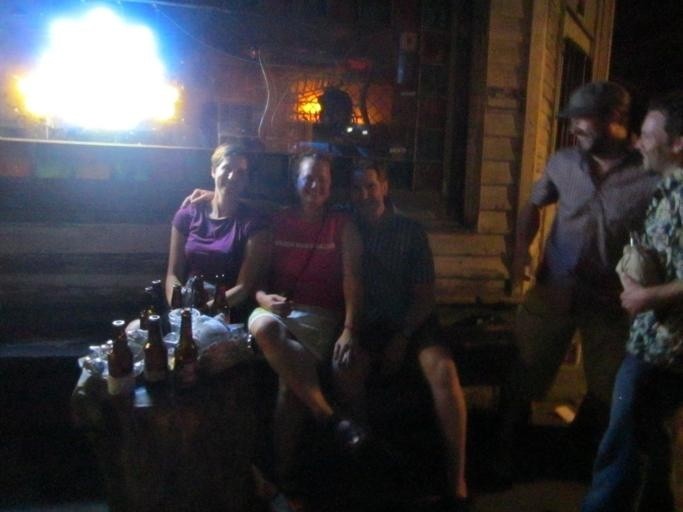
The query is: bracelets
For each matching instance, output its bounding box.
[393,330,410,352]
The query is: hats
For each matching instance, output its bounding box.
[555,81,630,120]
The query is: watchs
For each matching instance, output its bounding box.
[341,318,361,335]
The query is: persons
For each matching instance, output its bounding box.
[577,97,681,510]
[175,154,470,512]
[313,76,354,147]
[164,139,281,505]
[488,79,663,478]
[244,146,369,494]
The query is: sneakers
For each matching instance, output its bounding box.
[325,414,368,451]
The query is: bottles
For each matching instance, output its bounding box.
[106,273,230,399]
